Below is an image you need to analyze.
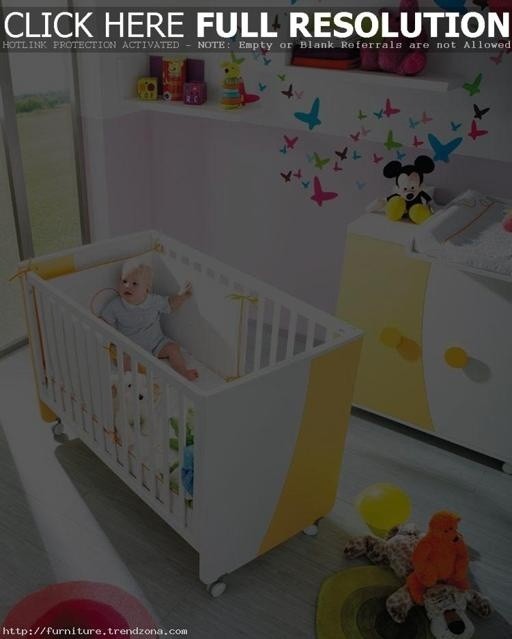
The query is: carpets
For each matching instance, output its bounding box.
[314,565,434,639]
[1,581,159,639]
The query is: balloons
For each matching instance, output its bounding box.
[357,481,413,532]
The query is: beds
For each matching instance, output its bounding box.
[15,230,366,598]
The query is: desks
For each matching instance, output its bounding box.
[337,200,511,475]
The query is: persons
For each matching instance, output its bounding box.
[100,257,200,383]
